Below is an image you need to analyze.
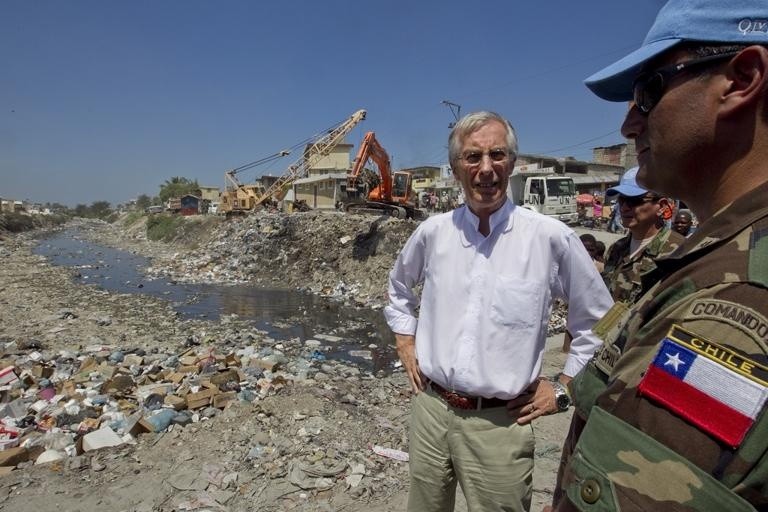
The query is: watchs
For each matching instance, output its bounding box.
[553,381,571,411]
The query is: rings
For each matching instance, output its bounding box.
[528,404,535,413]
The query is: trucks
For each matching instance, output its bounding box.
[506,169,579,225]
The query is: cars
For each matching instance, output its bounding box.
[211,202,226,215]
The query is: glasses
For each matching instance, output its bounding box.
[618,195,655,206]
[632,50,742,116]
[453,147,509,164]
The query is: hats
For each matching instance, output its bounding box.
[606,167,647,197]
[581,1,767,103]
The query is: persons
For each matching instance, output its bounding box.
[577,194,626,235]
[384,112,619,512]
[564,234,604,351]
[339,200,344,211]
[674,211,698,239]
[596,240,608,264]
[600,166,688,303]
[293,199,312,211]
[335,201,342,212]
[661,194,675,230]
[413,189,466,213]
[550,1,768,512]
[262,196,283,212]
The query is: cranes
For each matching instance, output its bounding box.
[216,108,366,221]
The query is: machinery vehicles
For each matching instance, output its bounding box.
[339,131,427,221]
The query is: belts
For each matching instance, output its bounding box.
[425,378,508,411]
